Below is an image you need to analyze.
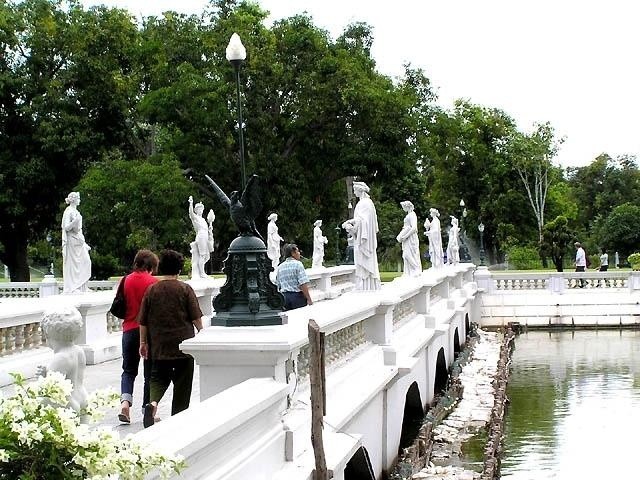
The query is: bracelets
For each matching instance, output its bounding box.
[141,342,146,344]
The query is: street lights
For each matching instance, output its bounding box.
[334,224,342,266]
[457,197,489,266]
[43,233,55,275]
[208,30,289,328]
[341,199,355,265]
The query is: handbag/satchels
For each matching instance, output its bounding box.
[110,277,127,320]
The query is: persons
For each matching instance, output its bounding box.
[115,249,161,425]
[341,180,382,290]
[267,213,285,265]
[274,243,313,310]
[35,305,90,418]
[423,207,444,269]
[61,191,93,294]
[136,250,205,428]
[445,218,460,267]
[310,220,327,267]
[188,194,214,276]
[396,201,422,278]
[579,246,589,288]
[571,241,586,288]
[595,247,610,288]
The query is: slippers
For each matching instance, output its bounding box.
[118,414,130,422]
[143,403,154,428]
